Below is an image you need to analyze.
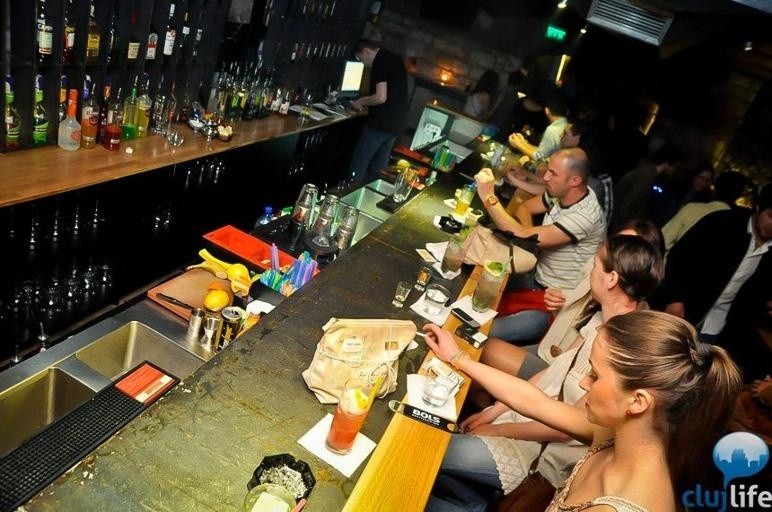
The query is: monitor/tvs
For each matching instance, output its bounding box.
[338,58,364,100]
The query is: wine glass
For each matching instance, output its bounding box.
[5,159,226,320]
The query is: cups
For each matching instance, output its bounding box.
[293,183,359,249]
[244,482,300,511]
[389,146,511,217]
[423,283,450,314]
[416,267,431,289]
[393,282,411,308]
[327,377,375,456]
[420,367,458,406]
[473,261,505,314]
[442,233,469,274]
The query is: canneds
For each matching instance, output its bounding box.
[213,310,242,354]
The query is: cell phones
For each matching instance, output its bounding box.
[450,307,481,330]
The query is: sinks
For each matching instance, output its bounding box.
[74,319,207,382]
[0,366,97,460]
[330,178,396,248]
[429,139,474,164]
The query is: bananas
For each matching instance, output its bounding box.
[185,260,227,279]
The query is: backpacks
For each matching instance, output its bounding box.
[497,473,557,512]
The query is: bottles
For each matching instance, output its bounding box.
[188,305,219,353]
[2,2,352,147]
[256,207,273,227]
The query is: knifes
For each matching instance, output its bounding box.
[156,291,194,313]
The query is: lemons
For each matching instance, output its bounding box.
[355,388,368,409]
[203,290,229,312]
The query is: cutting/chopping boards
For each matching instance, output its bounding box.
[147,268,252,327]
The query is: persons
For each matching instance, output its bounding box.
[350,38,407,190]
[421,64,772,512]
